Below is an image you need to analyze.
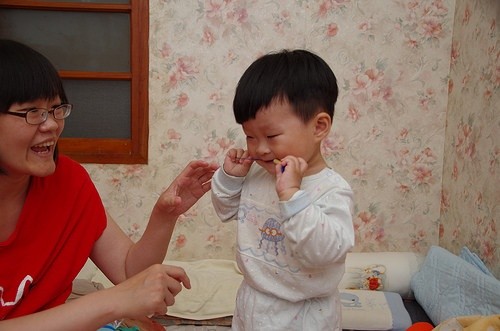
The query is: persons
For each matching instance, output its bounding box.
[211,50,354,331]
[0,39,221,331]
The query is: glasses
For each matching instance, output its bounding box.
[0,103,73,125]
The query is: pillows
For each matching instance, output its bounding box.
[338,288,412,331]
[344,251,426,295]
[148,260,247,317]
